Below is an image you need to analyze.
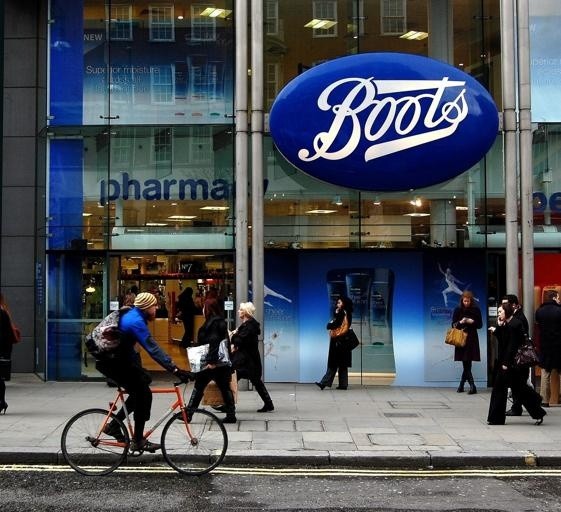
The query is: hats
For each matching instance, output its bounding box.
[133,292,158,309]
[462,291,474,298]
[240,302,256,318]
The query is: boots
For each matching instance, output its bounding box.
[220,391,236,424]
[173,389,204,423]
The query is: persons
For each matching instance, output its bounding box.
[0,292,15,414]
[96,292,194,452]
[212,302,274,412]
[438,262,480,307]
[452,291,483,395]
[315,297,352,390]
[177,287,197,348]
[502,294,529,417]
[248,280,292,307]
[487,303,548,425]
[174,299,237,423]
[536,290,561,407]
[264,328,281,370]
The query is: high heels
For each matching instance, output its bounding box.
[0,403,8,415]
[535,413,547,425]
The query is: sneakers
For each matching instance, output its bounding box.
[468,388,477,394]
[457,386,464,392]
[103,423,124,442]
[130,439,161,451]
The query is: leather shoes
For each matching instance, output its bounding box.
[505,410,516,416]
[315,382,325,390]
[257,401,274,412]
[211,404,225,413]
[336,386,347,390]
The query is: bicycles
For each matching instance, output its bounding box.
[61,378,228,475]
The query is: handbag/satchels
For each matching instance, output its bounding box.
[186,317,232,373]
[514,337,539,367]
[86,306,131,360]
[445,321,468,347]
[4,308,20,344]
[336,327,359,353]
